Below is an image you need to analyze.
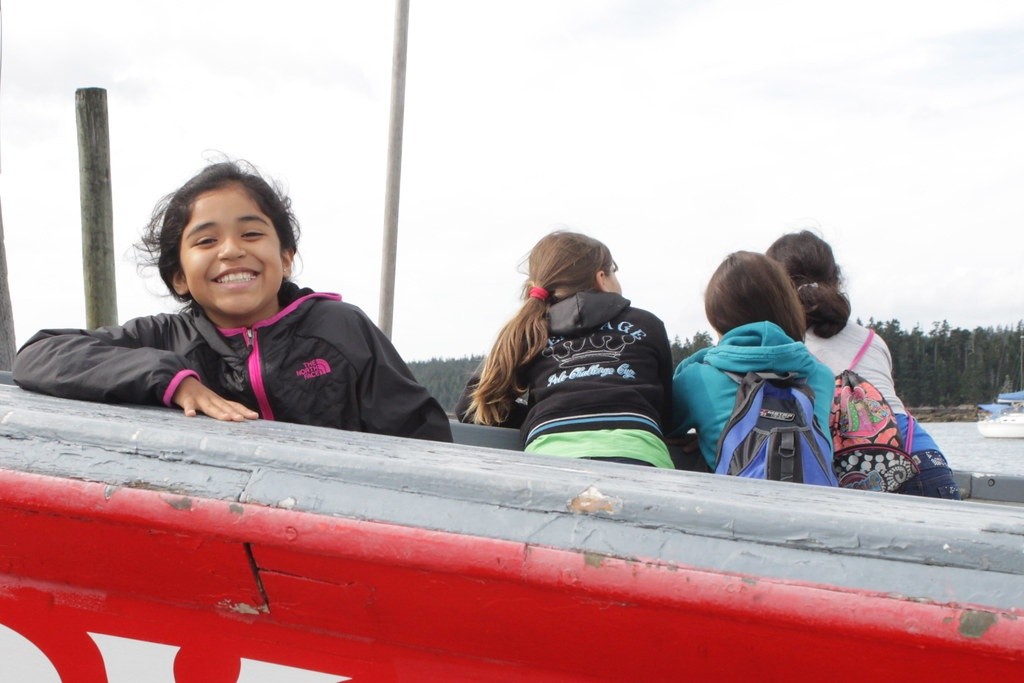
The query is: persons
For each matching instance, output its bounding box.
[666,251,839,486]
[767,230,964,504]
[458,232,676,468]
[11,163,455,444]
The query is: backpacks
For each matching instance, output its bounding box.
[829,328,920,493]
[702,361,839,488]
